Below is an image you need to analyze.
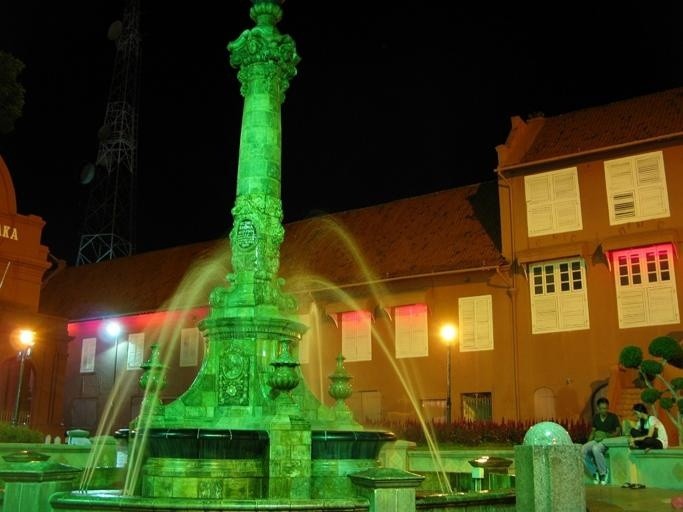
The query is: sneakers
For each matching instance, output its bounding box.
[593,469,608,485]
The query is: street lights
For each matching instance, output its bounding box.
[99,320,124,384]
[11,328,38,426]
[438,323,460,425]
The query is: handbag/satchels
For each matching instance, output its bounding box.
[595,431,606,441]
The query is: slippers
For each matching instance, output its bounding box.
[622,483,645,489]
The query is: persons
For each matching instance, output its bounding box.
[628,403,668,449]
[580,397,621,486]
